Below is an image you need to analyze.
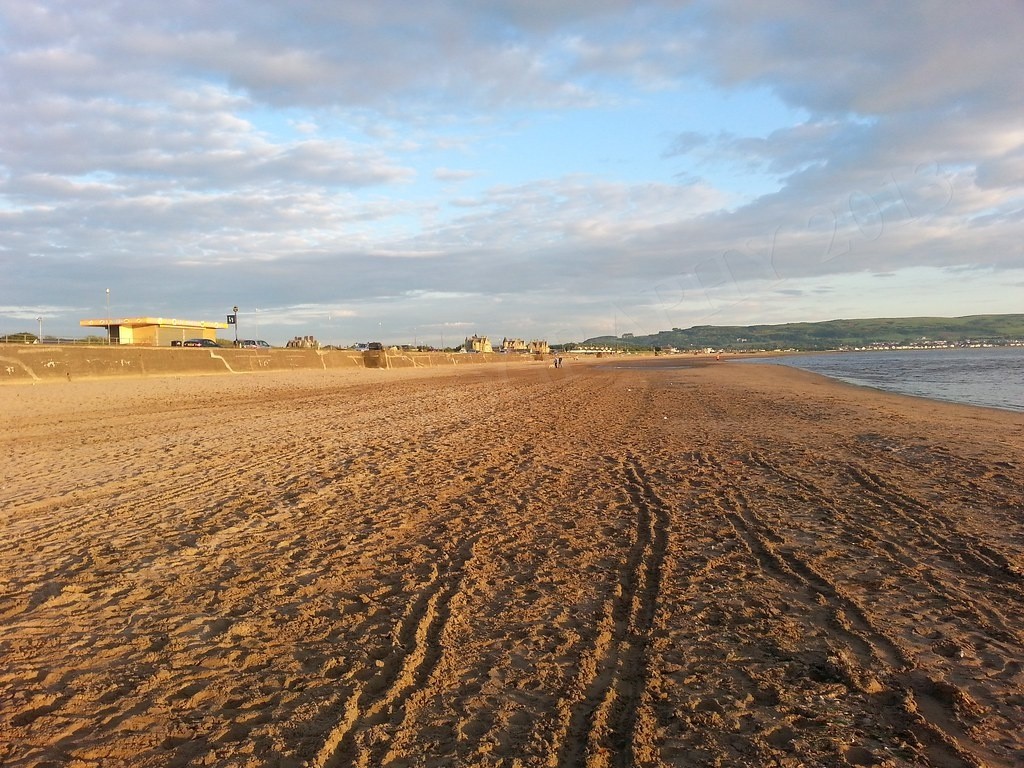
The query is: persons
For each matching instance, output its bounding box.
[716,355,719,361]
[553,356,562,368]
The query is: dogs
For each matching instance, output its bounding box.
[548,365,554,368]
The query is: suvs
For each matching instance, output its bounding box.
[352,341,382,353]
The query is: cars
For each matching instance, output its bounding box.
[468,347,506,356]
[242,340,270,349]
[185,339,223,348]
